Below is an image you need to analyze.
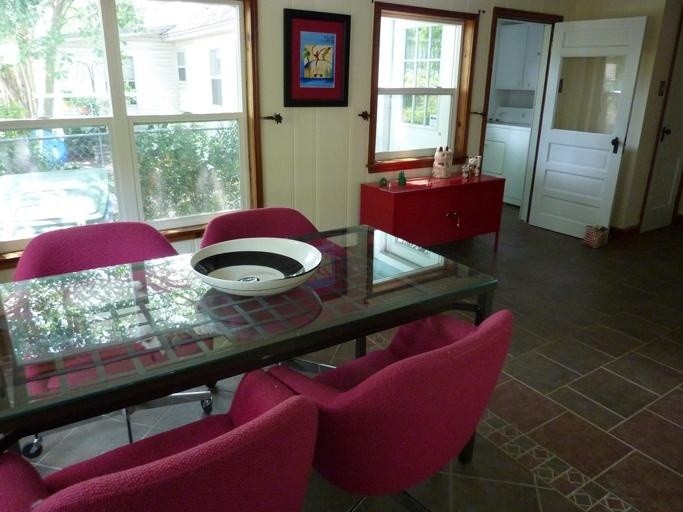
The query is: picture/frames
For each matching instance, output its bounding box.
[283,9,351,107]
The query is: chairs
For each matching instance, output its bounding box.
[0,207,511,512]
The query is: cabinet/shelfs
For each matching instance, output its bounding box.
[482,122,530,207]
[360,170,507,255]
[492,21,545,90]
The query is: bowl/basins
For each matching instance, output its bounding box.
[189,236,323,298]
